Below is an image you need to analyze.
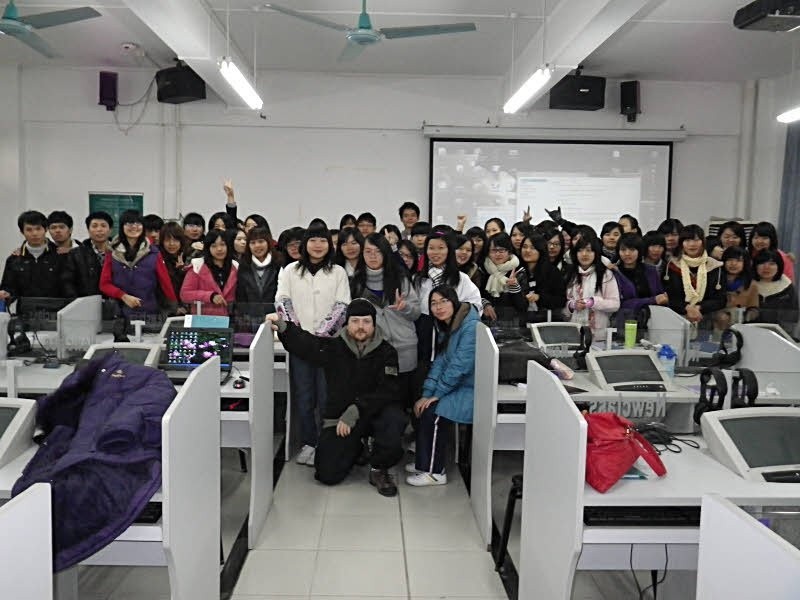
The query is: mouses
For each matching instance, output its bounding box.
[233,379,245,388]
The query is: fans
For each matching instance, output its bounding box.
[263,0,478,61]
[0,0,103,60]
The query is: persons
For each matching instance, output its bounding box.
[404,285,483,486]
[0,175,800,465]
[266,298,407,497]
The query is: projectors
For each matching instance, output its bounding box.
[733,0,800,34]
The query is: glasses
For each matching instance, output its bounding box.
[287,241,301,249]
[124,221,141,225]
[490,248,508,254]
[548,242,561,247]
[398,250,413,259]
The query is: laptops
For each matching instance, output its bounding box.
[157,328,235,386]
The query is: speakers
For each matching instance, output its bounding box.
[98,71,119,106]
[155,65,205,104]
[549,75,607,111]
[620,81,641,115]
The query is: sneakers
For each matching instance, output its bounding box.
[297,445,315,465]
[357,443,371,465]
[368,469,398,496]
[406,471,448,486]
[405,462,422,472]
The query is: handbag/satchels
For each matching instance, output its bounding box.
[582,414,666,494]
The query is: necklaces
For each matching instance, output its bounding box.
[211,260,224,287]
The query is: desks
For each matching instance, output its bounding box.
[476,309,800,600]
[0,293,301,600]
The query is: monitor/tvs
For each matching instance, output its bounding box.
[0,396,38,463]
[529,323,586,348]
[83,342,161,369]
[702,406,800,484]
[585,349,671,393]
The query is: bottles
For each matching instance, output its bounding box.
[625,319,637,348]
[657,344,677,388]
